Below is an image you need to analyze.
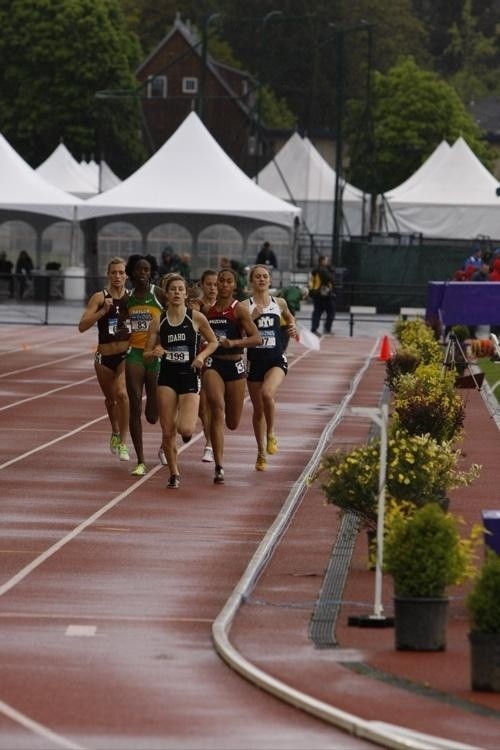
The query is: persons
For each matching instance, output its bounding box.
[125,248,309,317]
[451,245,500,282]
[191,269,219,462]
[117,254,166,477]
[1,249,34,299]
[77,257,132,461]
[255,240,278,269]
[198,268,261,485]
[308,255,337,338]
[239,264,298,471]
[142,273,220,489]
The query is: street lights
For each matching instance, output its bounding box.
[197,12,223,118]
[253,6,286,157]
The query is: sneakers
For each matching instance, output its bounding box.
[109,432,121,455]
[201,447,214,462]
[158,448,168,465]
[214,464,225,484]
[117,441,129,461]
[313,331,321,338]
[166,474,181,489]
[182,434,192,442]
[266,432,278,455]
[255,453,266,471]
[131,463,146,475]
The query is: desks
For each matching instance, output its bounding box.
[427,280,500,328]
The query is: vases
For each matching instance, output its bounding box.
[393,594,451,652]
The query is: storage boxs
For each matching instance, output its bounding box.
[481,508,500,554]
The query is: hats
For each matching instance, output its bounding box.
[163,246,173,255]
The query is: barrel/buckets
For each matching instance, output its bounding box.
[64,268,84,298]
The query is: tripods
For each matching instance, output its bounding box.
[442,343,481,390]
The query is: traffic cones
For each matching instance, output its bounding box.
[375,333,394,362]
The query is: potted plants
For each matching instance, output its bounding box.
[460,544,500,693]
